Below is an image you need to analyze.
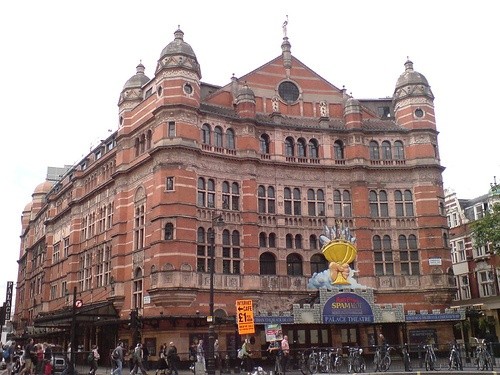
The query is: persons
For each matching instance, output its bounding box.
[128,343,151,375]
[190,338,207,372]
[213,339,220,358]
[88,345,100,375]
[0,338,53,375]
[281,335,289,372]
[111,342,126,375]
[155,343,168,375]
[267,340,281,371]
[237,338,253,371]
[167,342,180,375]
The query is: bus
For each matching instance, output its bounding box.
[49,344,67,372]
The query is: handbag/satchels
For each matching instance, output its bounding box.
[32,354,38,364]
[4,347,10,358]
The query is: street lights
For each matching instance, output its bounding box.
[208,211,226,375]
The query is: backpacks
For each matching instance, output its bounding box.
[88,351,94,361]
[112,349,119,360]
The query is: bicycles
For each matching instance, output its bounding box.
[274,345,395,375]
[447,342,465,371]
[423,343,437,371]
[472,337,494,372]
[398,344,413,372]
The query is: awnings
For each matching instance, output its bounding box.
[34,302,119,327]
